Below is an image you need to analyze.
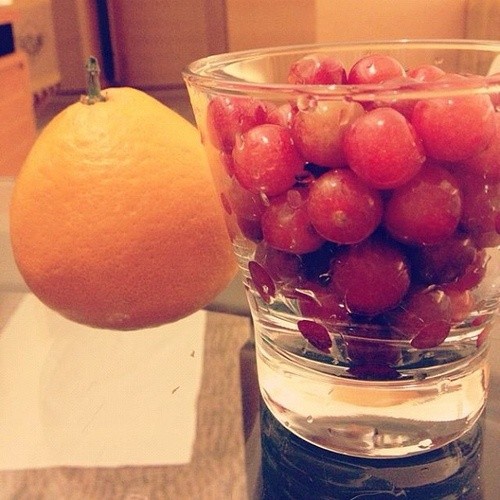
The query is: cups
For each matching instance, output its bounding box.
[183,39,500,459]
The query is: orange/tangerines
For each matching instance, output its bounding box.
[10,54,238,332]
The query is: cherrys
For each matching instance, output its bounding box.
[202,52,499,376]
